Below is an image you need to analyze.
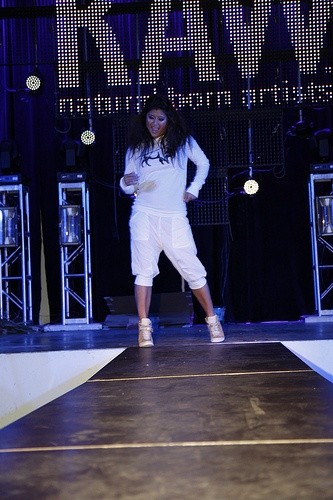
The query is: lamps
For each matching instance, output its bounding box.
[242,175,259,194]
[26,68,44,90]
[80,127,97,145]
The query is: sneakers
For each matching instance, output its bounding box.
[205,313,225,342]
[138,317,154,348]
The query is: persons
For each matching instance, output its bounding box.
[119,94,225,347]
